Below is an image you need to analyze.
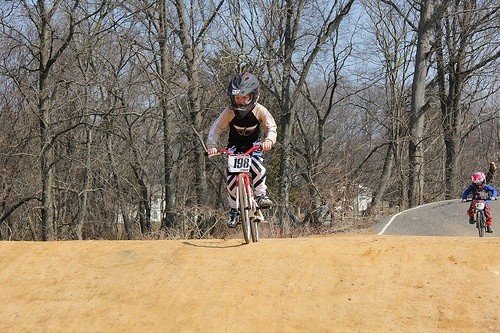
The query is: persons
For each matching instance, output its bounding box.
[206,72,277,228]
[461,172,497,233]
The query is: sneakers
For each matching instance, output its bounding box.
[469,218,475,224]
[487,225,494,232]
[254,194,273,207]
[227,208,241,228]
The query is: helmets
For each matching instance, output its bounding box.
[470,171,486,191]
[227,72,260,120]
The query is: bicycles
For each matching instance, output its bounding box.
[203,134,275,244]
[461,196,497,237]
